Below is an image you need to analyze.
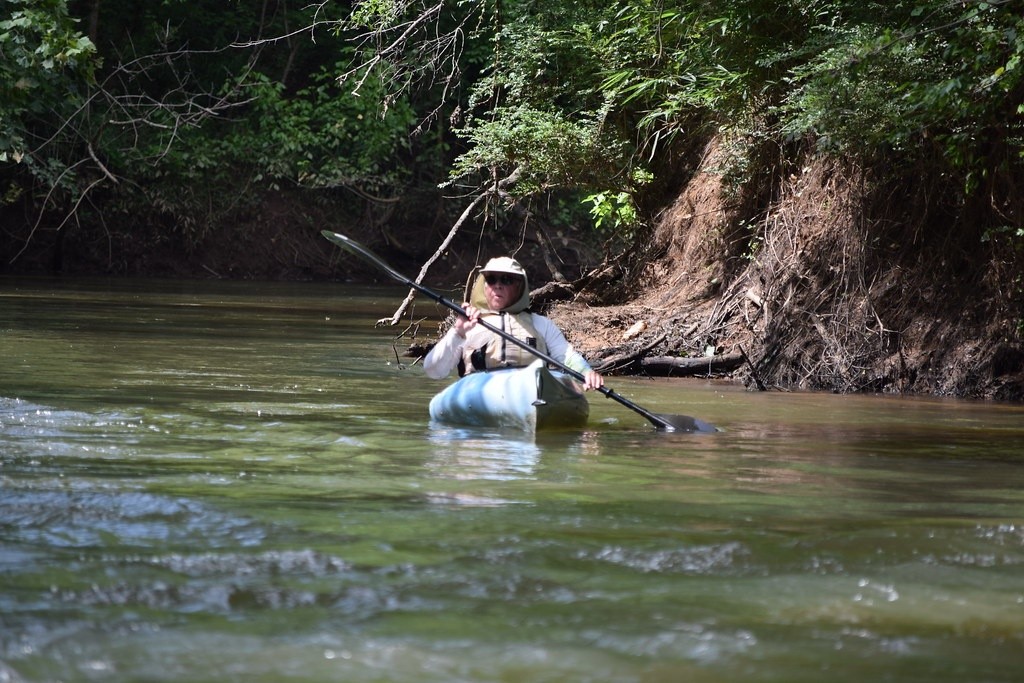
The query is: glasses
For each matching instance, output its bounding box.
[485,275,518,285]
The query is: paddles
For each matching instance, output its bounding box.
[320,228,721,435]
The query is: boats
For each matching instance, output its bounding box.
[427,357,590,440]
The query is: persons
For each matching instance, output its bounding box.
[424,257,605,391]
